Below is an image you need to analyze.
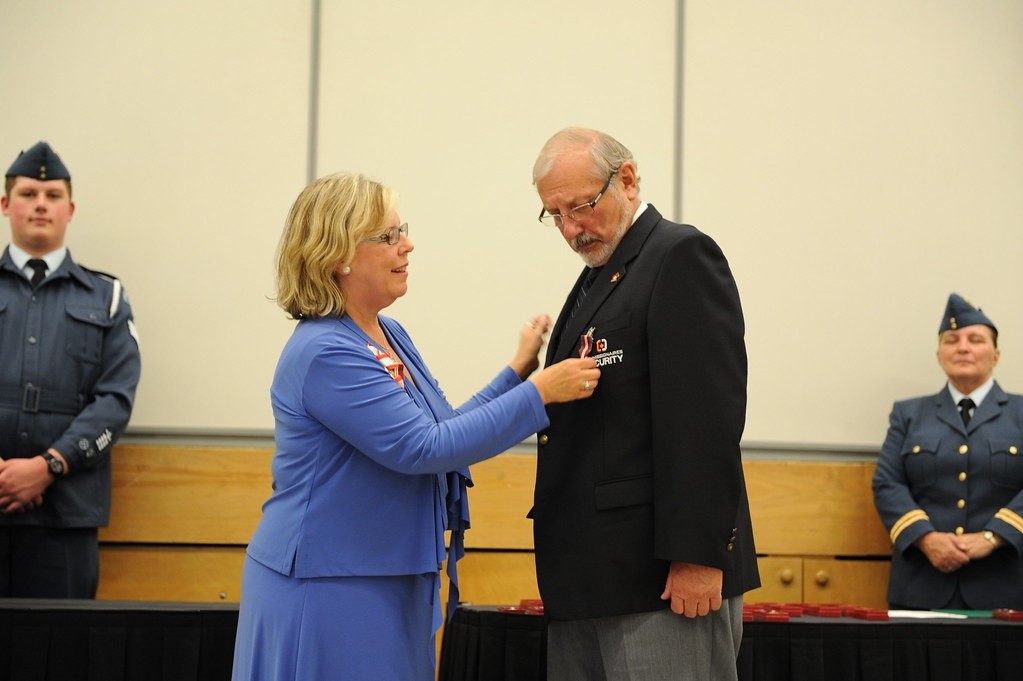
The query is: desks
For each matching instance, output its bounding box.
[0,596,1023,681]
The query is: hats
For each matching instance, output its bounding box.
[5,141,71,180]
[938,293,997,335]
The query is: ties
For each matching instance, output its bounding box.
[958,399,974,428]
[558,264,604,346]
[28,258,49,287]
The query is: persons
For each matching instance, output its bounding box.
[232,171,600,681]
[525,126,761,681]
[0,140,141,599]
[872,293,1023,611]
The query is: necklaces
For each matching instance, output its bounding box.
[354,322,397,362]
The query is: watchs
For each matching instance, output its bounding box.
[983,530,997,547]
[40,449,64,479]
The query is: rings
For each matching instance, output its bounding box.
[585,381,589,390]
[526,321,534,327]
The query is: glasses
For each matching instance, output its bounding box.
[360,222,408,244]
[538,165,621,228]
